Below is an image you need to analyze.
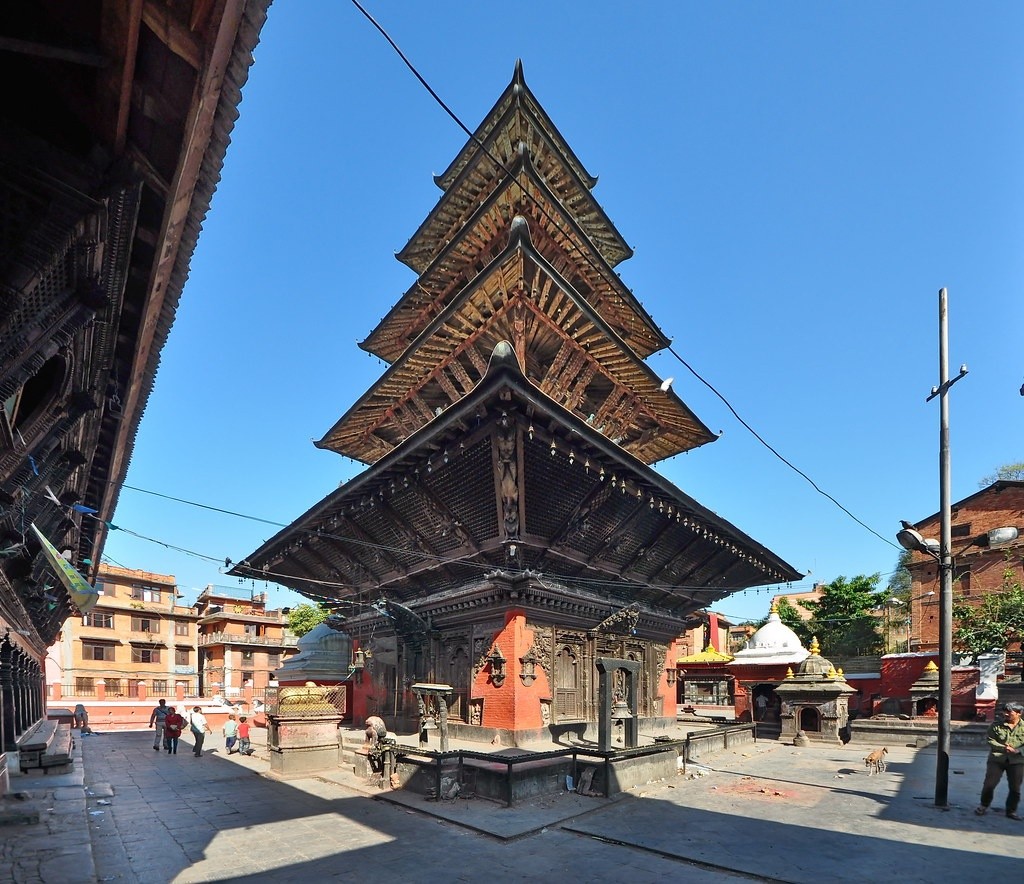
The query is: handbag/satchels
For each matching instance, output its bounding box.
[181,717,189,729]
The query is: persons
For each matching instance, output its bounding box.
[974,701,1024,820]
[222,715,238,754]
[756,694,768,716]
[164,707,184,755]
[149,699,169,751]
[235,716,250,755]
[190,706,212,758]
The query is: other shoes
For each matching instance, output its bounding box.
[153,745,159,750]
[975,805,989,815]
[195,754,203,757]
[242,750,248,755]
[225,747,230,755]
[1007,812,1022,820]
[173,750,176,754]
[193,749,196,752]
[164,747,172,750]
[169,751,172,754]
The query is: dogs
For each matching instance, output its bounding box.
[863,747,889,777]
